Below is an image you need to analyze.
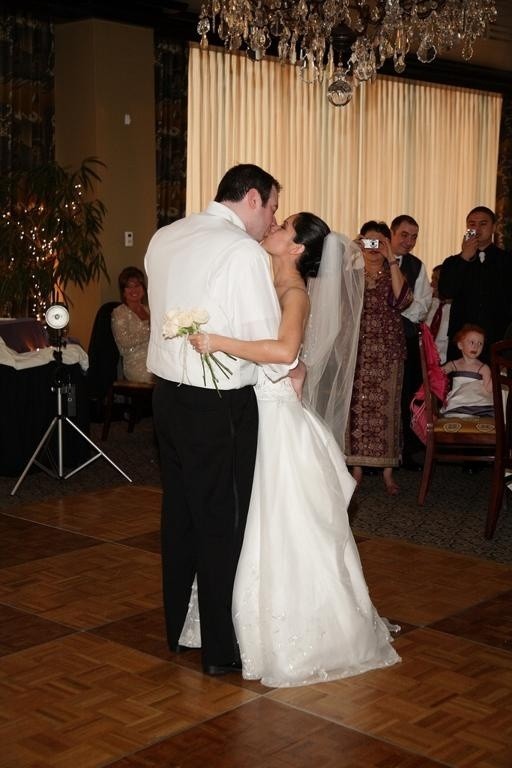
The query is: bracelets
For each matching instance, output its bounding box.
[389,259,400,267]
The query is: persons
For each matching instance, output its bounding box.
[421,264,454,470]
[341,218,415,496]
[109,265,163,387]
[187,210,404,690]
[433,204,510,476]
[438,321,501,421]
[138,162,308,678]
[361,214,435,474]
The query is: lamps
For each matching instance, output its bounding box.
[196,1,498,107]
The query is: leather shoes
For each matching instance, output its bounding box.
[202,652,243,676]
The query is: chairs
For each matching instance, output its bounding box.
[101,302,153,442]
[418,320,512,541]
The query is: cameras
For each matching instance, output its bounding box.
[361,238,379,248]
[466,228,476,241]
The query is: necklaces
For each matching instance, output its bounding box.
[363,266,385,281]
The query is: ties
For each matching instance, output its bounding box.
[478,251,486,264]
[430,302,447,341]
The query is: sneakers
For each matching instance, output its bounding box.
[385,478,400,494]
[351,474,362,493]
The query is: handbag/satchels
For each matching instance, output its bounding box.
[410,399,438,445]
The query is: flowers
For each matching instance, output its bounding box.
[162,306,237,401]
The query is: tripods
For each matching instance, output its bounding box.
[10,329,132,495]
